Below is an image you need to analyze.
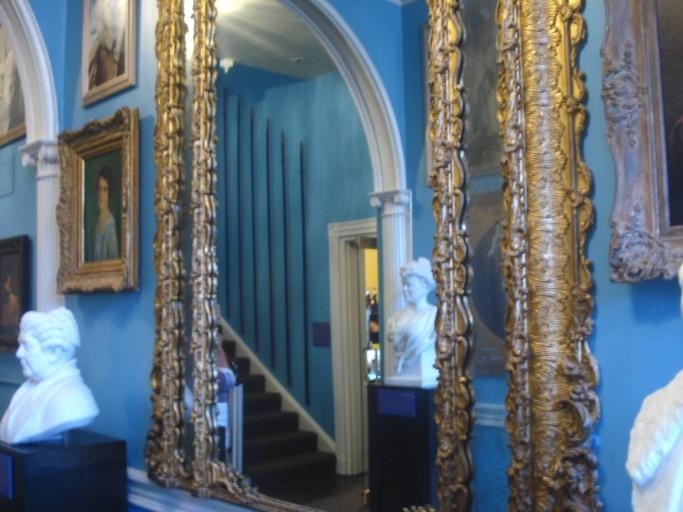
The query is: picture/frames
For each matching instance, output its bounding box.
[56,104,140,293]
[80,0,136,106]
[598,0,683,281]
[0,234,29,346]
[0,22,26,146]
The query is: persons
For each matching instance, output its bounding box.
[386,255,437,370]
[186,329,235,462]
[369,292,380,342]
[365,290,372,344]
[0,304,100,444]
[90,164,121,260]
